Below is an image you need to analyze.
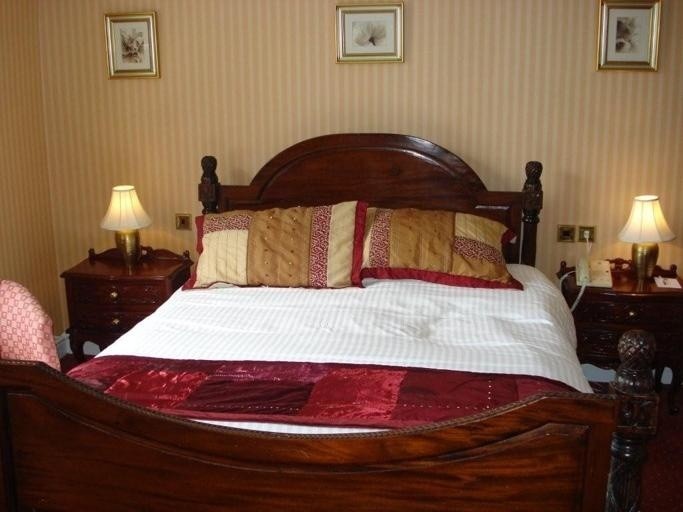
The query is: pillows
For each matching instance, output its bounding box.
[183,199,369,290]
[361,207,526,290]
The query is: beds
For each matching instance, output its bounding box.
[0,133,660,512]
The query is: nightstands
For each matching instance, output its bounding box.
[560,259,683,415]
[60,248,194,363]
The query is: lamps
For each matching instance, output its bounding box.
[99,183,152,267]
[617,196,673,277]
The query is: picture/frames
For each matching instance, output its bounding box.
[334,2,404,63]
[596,0,663,71]
[103,11,160,81]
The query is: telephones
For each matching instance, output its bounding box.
[576,255,613,288]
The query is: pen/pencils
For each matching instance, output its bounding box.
[658,275,666,285]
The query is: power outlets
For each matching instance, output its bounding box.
[578,224,594,242]
